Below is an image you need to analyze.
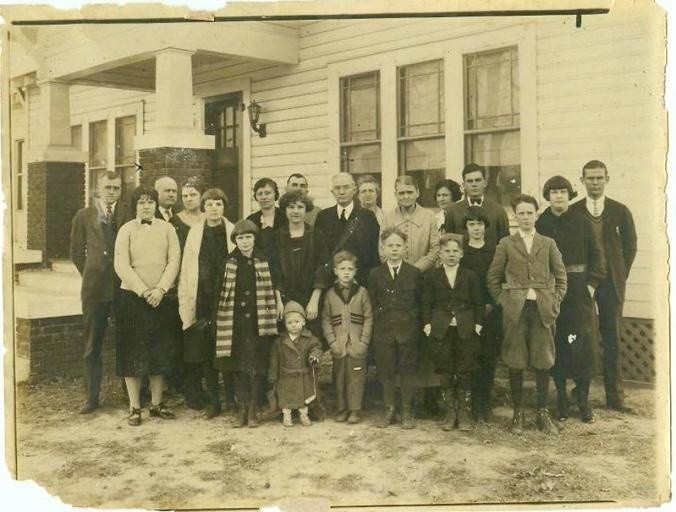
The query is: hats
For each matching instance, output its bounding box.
[283,299,307,317]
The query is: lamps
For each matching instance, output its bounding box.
[246,98,267,138]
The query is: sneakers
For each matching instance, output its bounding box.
[536,408,561,436]
[335,409,347,422]
[283,414,293,427]
[348,411,360,423]
[442,409,457,431]
[299,414,311,426]
[458,408,476,431]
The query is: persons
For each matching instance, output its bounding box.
[566,160,640,416]
[486,193,567,435]
[70,170,147,415]
[535,175,605,423]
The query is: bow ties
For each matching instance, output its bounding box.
[471,197,481,205]
[141,219,152,225]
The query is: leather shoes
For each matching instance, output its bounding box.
[226,402,238,412]
[149,402,175,419]
[79,398,100,414]
[233,415,247,428]
[608,403,638,415]
[247,415,259,427]
[559,401,568,422]
[185,394,203,410]
[510,409,527,435]
[577,399,595,424]
[129,402,141,426]
[400,407,416,429]
[205,400,221,418]
[377,409,396,427]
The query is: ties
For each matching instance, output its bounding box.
[106,205,113,224]
[593,199,599,217]
[340,208,346,221]
[164,209,171,218]
[393,266,399,280]
